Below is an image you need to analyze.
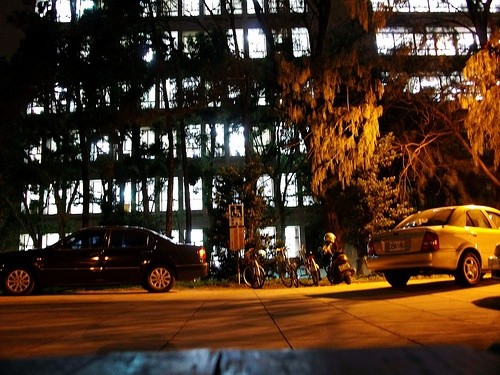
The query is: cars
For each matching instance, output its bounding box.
[365,204,500,288]
[1,226,207,297]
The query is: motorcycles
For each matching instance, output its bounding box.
[316,233,356,285]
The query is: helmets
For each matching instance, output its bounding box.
[323,232,336,243]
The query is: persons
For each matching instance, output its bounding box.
[321,232,337,270]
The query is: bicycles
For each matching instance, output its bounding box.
[271,246,299,287]
[296,245,321,287]
[242,247,266,289]
